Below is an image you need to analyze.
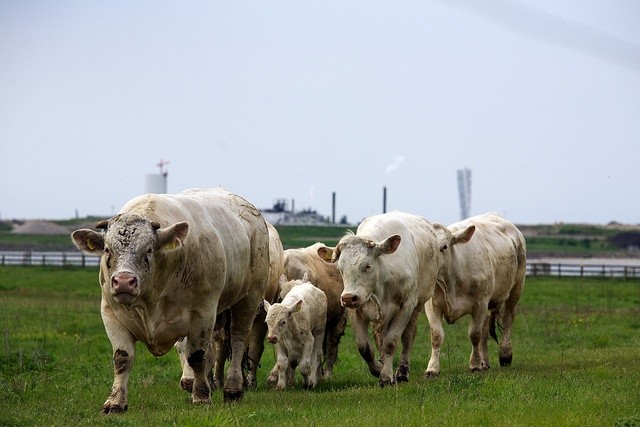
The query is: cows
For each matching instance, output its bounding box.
[317,210,439,388]
[282,240,351,382]
[70,187,269,416]
[423,213,527,381]
[174,220,284,398]
[263,281,327,391]
